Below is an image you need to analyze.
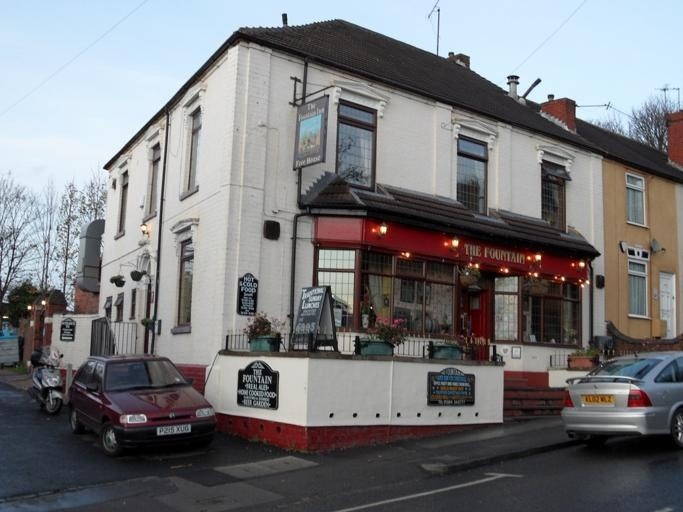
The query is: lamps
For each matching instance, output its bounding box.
[575,259,585,272]
[447,236,458,252]
[376,221,388,238]
[530,251,542,266]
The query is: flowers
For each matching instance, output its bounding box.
[242,310,275,341]
[373,316,408,343]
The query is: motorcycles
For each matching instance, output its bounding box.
[27,346,63,415]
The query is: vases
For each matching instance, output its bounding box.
[248,334,281,352]
[387,334,397,345]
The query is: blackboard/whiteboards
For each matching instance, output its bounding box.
[291,286,328,344]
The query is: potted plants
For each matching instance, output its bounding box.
[456,263,480,288]
[568,346,602,370]
[523,275,549,297]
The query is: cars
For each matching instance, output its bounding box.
[68,354,217,457]
[561,351,683,448]
